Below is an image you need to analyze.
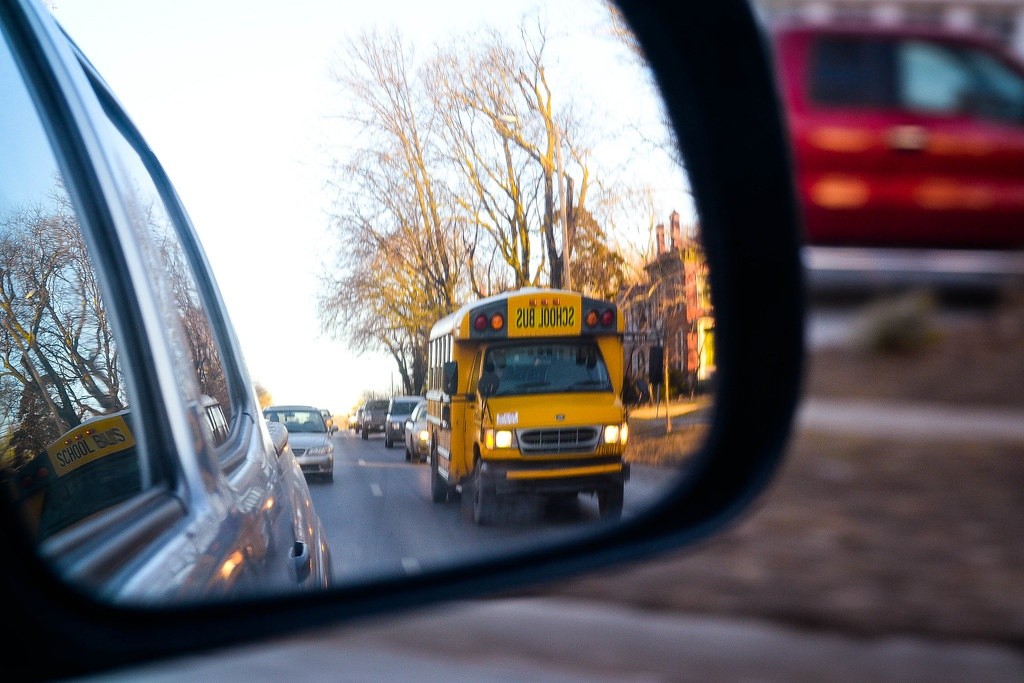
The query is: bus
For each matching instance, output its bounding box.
[422,285,649,528]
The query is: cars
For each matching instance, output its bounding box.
[403,399,431,461]
[382,395,425,449]
[768,26,1024,244]
[320,411,334,434]
[261,405,334,480]
[0,0,334,605]
[346,400,390,442]
